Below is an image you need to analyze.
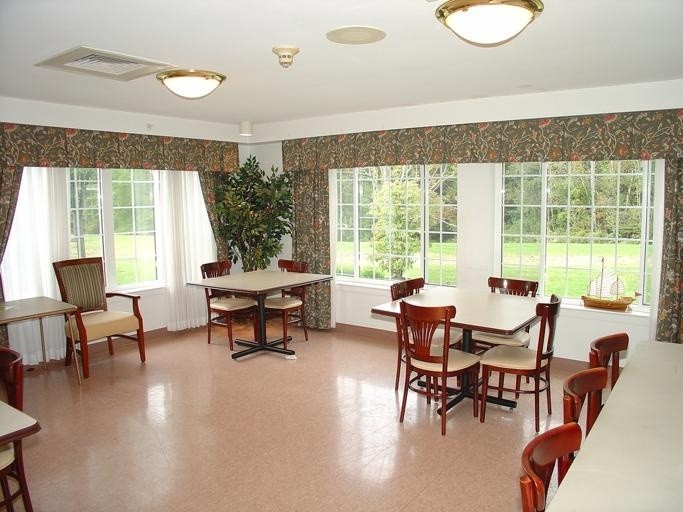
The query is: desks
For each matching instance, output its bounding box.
[0,399,40,447]
[183,270,334,361]
[371,288,541,414]
[542,338,682,512]
[0,295,81,387]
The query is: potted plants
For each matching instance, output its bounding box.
[210,154,296,320]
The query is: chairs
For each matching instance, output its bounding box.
[262,259,309,351]
[475,277,539,398]
[199,259,259,350]
[518,421,582,512]
[589,332,629,405]
[398,299,481,435]
[50,257,145,379]
[0,347,25,476]
[389,277,461,401]
[479,294,562,434]
[562,367,607,458]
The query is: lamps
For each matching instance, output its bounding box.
[156,69,227,100]
[238,120,253,137]
[434,0,545,47]
